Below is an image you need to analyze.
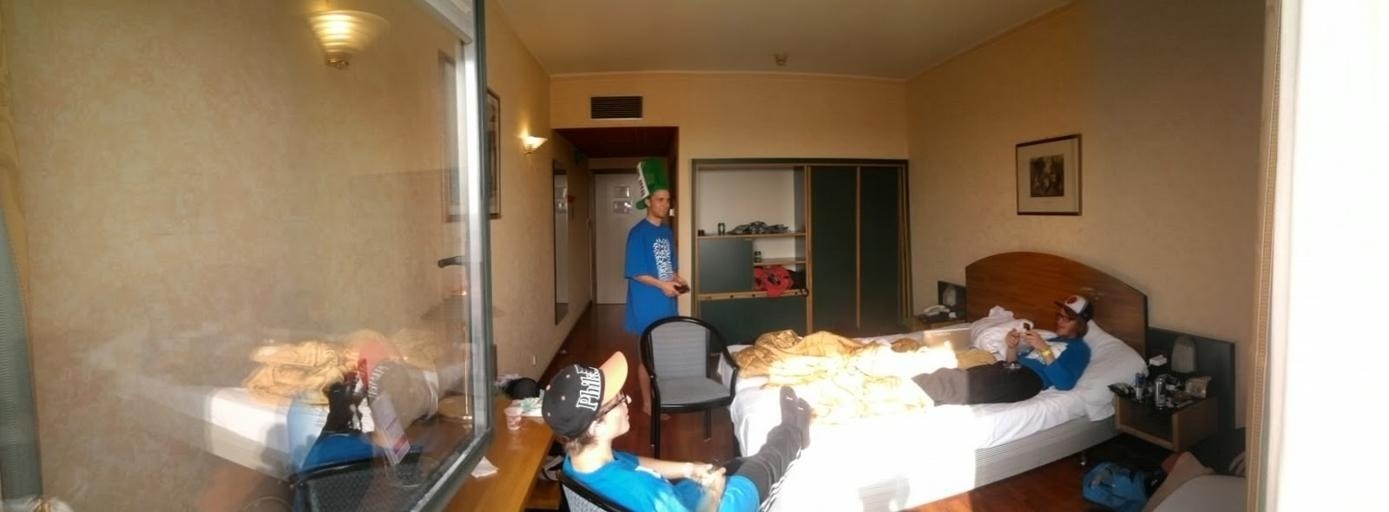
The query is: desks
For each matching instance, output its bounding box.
[434,387,560,510]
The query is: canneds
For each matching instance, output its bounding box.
[1154,377,1167,411]
[1135,372,1145,402]
[718,222,725,236]
[754,251,762,264]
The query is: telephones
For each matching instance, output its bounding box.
[923,305,950,317]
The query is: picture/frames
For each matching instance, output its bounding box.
[439,49,503,222]
[1016,130,1085,217]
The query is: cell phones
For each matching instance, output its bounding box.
[1024,323,1030,336]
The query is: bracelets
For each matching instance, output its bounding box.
[685,464,692,479]
[1041,346,1053,360]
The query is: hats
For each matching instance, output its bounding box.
[542,350,629,441]
[1054,295,1093,321]
[636,159,670,209]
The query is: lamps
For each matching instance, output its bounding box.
[302,2,392,74]
[518,133,549,158]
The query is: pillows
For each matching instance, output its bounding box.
[1055,313,1144,420]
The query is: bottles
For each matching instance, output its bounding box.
[1153,376,1168,410]
[754,249,762,263]
[1135,368,1146,402]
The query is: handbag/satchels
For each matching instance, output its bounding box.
[787,270,806,289]
[1081,461,1167,512]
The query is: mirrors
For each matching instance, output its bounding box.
[555,159,571,322]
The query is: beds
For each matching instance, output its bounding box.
[95,306,436,481]
[727,251,1149,510]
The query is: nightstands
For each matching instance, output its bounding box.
[1109,324,1237,488]
[916,276,967,328]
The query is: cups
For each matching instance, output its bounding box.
[718,222,725,234]
[503,406,525,432]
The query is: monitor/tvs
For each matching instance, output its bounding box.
[0,0,501,512]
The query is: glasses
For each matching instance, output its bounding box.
[1054,312,1075,323]
[596,389,628,419]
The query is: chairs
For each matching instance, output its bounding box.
[559,474,641,510]
[284,454,436,512]
[640,317,735,462]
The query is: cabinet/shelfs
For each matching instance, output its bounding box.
[691,159,812,348]
[804,157,908,336]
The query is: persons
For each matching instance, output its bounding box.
[911,289,1094,405]
[623,189,687,410]
[542,348,810,512]
